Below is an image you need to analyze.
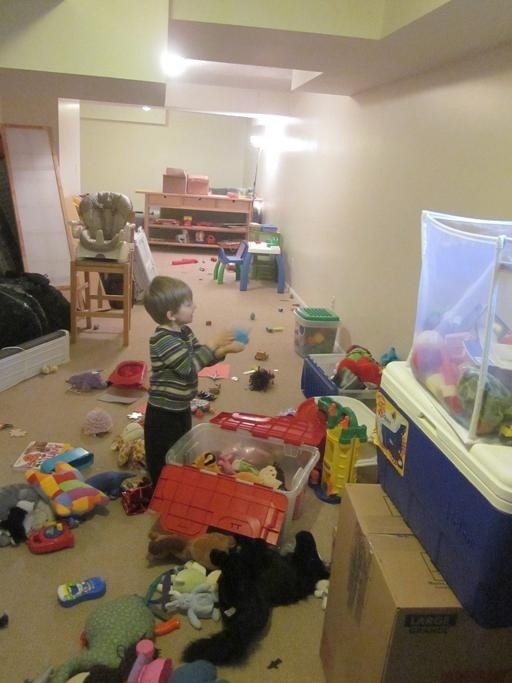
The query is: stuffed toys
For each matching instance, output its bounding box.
[45,595,217,683]
[80,408,150,499]
[164,526,331,668]
[0,341,400,682]
[1,484,55,548]
[332,346,400,389]
[193,451,287,493]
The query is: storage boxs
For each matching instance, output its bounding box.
[187,176,210,195]
[292,307,342,359]
[300,351,382,411]
[248,230,281,265]
[375,360,512,629]
[319,482,511,683]
[163,168,188,193]
[150,411,324,548]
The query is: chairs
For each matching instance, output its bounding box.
[213,240,247,285]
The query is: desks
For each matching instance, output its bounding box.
[240,243,285,294]
[135,190,254,252]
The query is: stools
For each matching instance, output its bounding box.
[69,259,135,346]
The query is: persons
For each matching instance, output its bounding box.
[140,277,244,486]
